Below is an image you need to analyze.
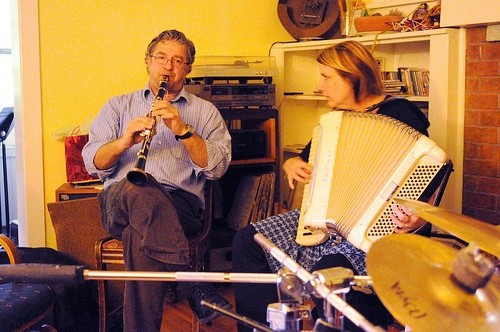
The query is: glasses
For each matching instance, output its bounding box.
[150,54,189,66]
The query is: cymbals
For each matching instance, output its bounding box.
[366,232,500,332]
[392,197,500,259]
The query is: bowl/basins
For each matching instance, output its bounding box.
[354,15,401,32]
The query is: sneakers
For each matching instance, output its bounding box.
[185,282,232,322]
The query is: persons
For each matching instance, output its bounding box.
[81,30,232,332]
[232,41,437,332]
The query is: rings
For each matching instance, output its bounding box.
[405,224,408,228]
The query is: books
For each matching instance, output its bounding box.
[227,171,275,231]
[381,67,428,97]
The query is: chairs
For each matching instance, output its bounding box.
[95,84,213,332]
[0,233,73,332]
[341,159,455,332]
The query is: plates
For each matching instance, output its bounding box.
[356,31,399,36]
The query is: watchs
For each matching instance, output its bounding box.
[175,124,194,141]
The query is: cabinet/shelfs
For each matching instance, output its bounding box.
[273,28,459,212]
[207,118,278,272]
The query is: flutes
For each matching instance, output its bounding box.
[127,76,169,187]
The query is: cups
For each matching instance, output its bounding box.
[339,12,354,37]
